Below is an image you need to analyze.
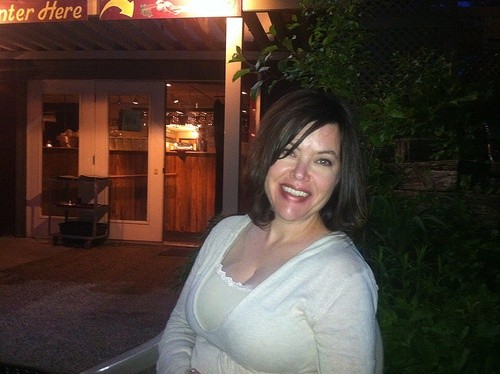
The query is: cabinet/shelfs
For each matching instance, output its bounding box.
[45,173,111,249]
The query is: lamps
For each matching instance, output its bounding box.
[164,113,214,126]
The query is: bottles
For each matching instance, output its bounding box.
[109,137,148,150]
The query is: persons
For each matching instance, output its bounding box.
[151,86,387,373]
